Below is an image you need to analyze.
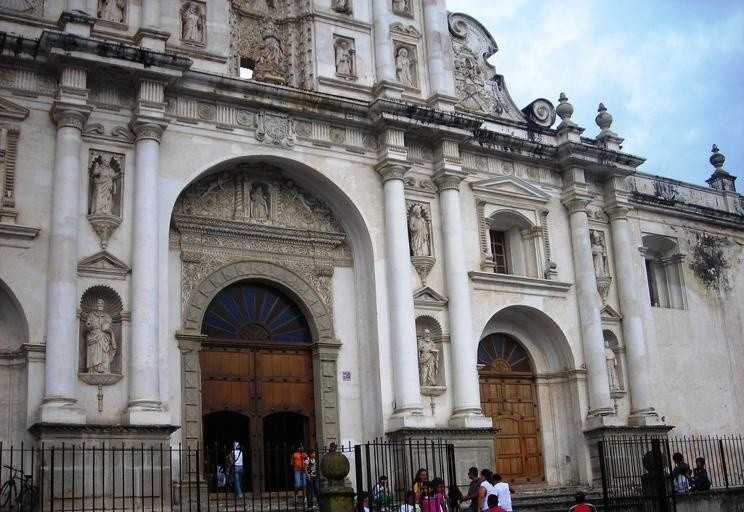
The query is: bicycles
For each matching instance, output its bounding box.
[0,464,40,512]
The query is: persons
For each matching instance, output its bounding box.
[289,445,305,498]
[186,2,207,42]
[251,186,268,221]
[301,451,322,508]
[640,440,720,505]
[255,12,288,68]
[591,227,609,280]
[92,152,121,214]
[85,297,118,373]
[356,464,515,511]
[228,440,246,497]
[332,0,427,88]
[570,493,594,511]
[602,338,622,391]
[409,205,431,258]
[418,329,440,386]
